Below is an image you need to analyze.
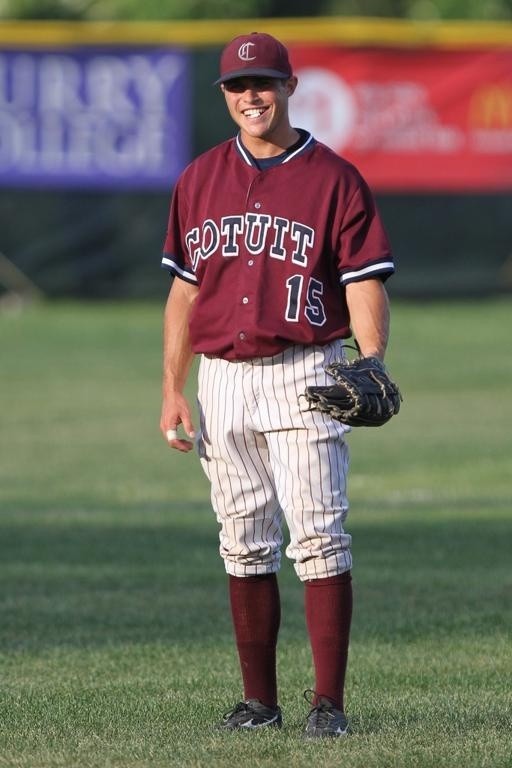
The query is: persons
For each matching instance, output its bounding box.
[157,34,400,745]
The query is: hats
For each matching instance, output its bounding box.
[210,31,295,88]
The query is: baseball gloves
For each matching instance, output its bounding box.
[303,355,402,426]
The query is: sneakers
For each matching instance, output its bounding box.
[295,689,353,742]
[211,697,284,733]
